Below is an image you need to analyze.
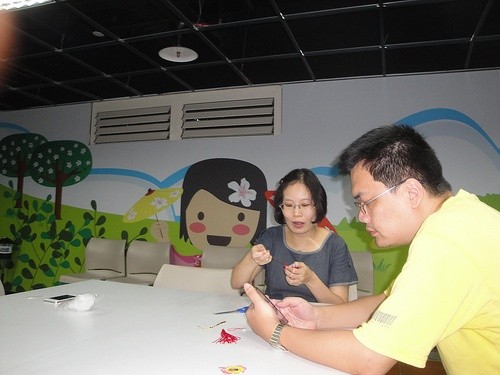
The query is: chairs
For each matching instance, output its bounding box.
[58,236,374,301]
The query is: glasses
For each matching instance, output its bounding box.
[354,178,420,214]
[278,202,314,215]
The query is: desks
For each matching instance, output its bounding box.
[0,279,353,375]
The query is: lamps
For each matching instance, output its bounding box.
[158,36,199,62]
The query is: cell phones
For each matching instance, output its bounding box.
[254,286,288,325]
[44,294,77,303]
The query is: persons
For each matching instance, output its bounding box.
[231,169,359,304]
[244,125,500,375]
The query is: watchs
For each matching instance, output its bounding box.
[269,324,285,351]
